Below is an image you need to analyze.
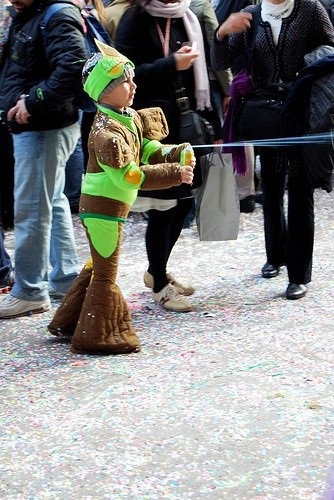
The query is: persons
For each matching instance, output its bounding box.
[214,0,334,300]
[0,0,334,230]
[115,0,224,313]
[47,52,197,354]
[0,226,18,286]
[0,1,88,321]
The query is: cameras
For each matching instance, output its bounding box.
[182,41,197,53]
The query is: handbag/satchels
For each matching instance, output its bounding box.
[178,104,215,155]
[231,82,297,140]
[194,146,240,241]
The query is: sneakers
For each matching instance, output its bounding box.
[144,268,195,295]
[152,282,194,312]
[47,279,66,298]
[0,293,52,319]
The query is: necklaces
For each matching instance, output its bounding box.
[263,1,293,21]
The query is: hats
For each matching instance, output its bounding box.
[82,53,135,100]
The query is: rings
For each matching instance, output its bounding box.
[15,112,21,116]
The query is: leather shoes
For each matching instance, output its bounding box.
[261,261,281,277]
[286,282,308,300]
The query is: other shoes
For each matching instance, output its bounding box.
[254,191,264,203]
[238,194,255,214]
